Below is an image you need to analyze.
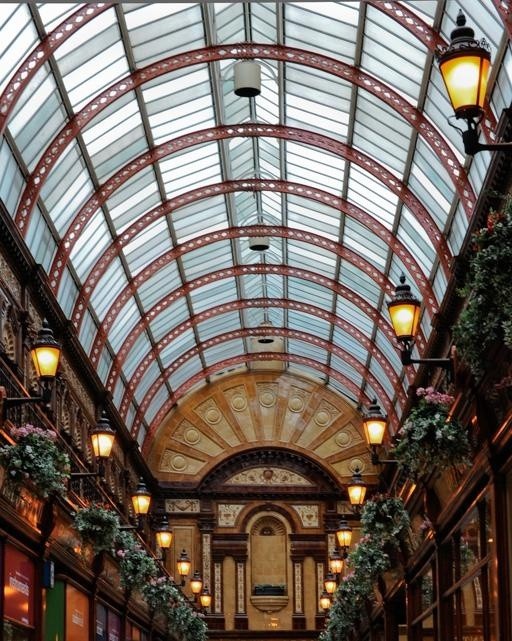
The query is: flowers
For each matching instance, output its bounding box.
[68,499,125,549]
[0,422,74,503]
[115,543,175,609]
[393,386,476,488]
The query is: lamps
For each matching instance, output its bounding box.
[0,318,67,406]
[183,569,204,604]
[319,397,399,612]
[64,408,117,484]
[232,58,261,99]
[115,474,157,532]
[385,271,460,369]
[194,585,213,617]
[248,236,270,251]
[434,9,511,157]
[170,548,193,589]
[140,513,178,563]
